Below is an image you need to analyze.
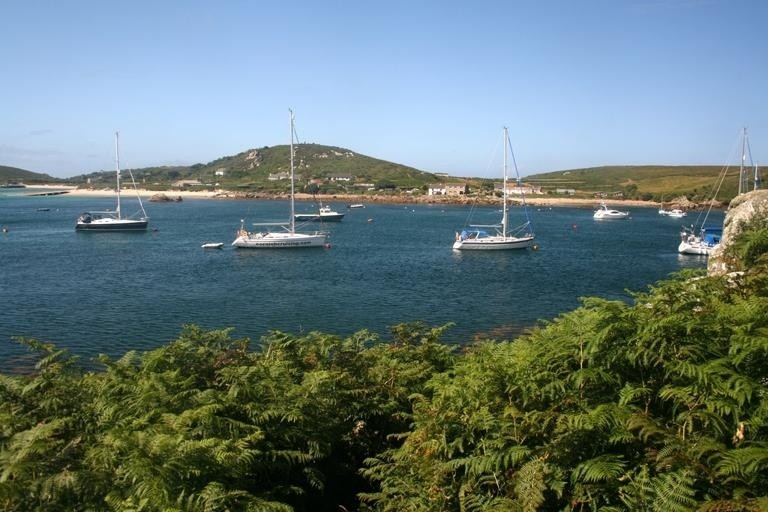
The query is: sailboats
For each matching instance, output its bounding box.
[677,127,760,258]
[232,108,329,249]
[75,131,149,232]
[452,126,537,251]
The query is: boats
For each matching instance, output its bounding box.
[658,206,688,218]
[294,206,346,222]
[595,206,630,220]
